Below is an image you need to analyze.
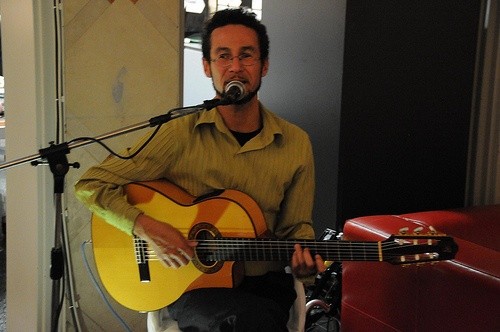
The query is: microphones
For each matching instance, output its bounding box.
[224,80,246,104]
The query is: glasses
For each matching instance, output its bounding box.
[205,54,260,67]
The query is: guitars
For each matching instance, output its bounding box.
[91,179,459,313]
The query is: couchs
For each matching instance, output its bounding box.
[342,205,500,332]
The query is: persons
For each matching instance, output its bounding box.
[75,8,327,332]
